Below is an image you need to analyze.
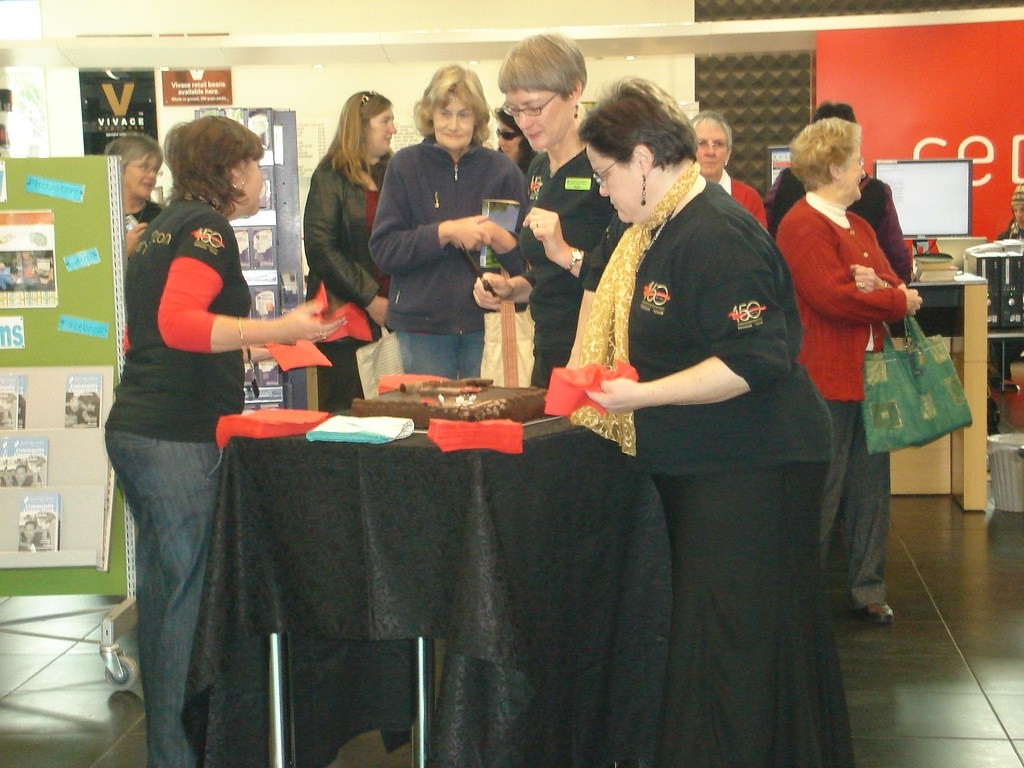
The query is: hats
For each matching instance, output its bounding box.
[1011,182,1024,207]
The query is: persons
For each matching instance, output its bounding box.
[18,511,56,552]
[489,101,538,174]
[303,90,397,415]
[105,133,168,262]
[369,64,531,379]
[993,182,1024,379]
[0,465,33,487]
[565,74,856,768]
[66,392,100,427]
[776,117,923,623]
[763,102,915,287]
[473,33,616,390]
[0,252,54,291]
[690,110,768,232]
[107,121,349,768]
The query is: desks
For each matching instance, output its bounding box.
[889,270,988,512]
[176,391,676,768]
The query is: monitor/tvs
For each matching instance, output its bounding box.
[872,158,972,262]
[767,146,791,193]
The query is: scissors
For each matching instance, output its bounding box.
[457,242,498,299]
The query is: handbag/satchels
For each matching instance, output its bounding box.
[860,314,974,454]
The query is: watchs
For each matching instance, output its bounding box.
[566,249,583,272]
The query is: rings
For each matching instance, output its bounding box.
[536,220,539,228]
[859,281,865,288]
[320,332,326,339]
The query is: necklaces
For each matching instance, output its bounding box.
[125,199,147,223]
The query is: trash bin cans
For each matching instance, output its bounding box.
[987,433,1024,512]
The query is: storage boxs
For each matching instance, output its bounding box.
[964,239,1024,332]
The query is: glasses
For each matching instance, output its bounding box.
[128,161,163,175]
[592,154,624,188]
[501,92,558,117]
[698,138,730,151]
[360,89,377,108]
[496,128,524,140]
[835,156,864,171]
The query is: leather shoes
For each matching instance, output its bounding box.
[856,602,893,624]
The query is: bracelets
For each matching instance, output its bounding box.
[883,280,888,288]
[237,316,246,346]
